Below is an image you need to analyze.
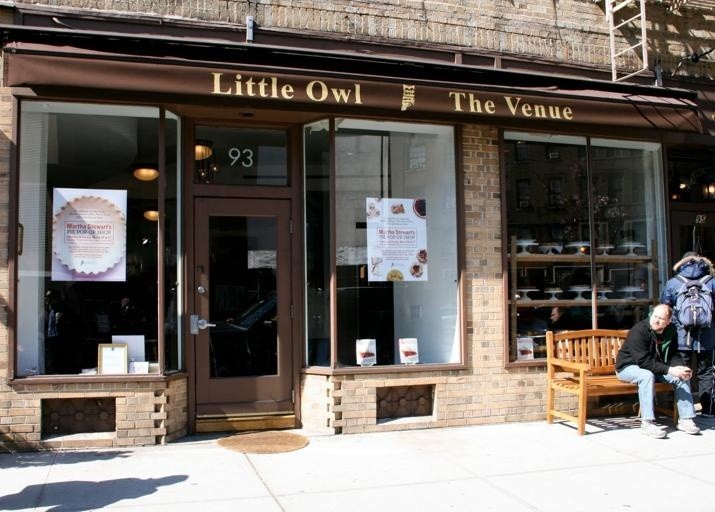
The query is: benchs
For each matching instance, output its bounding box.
[542,322,683,433]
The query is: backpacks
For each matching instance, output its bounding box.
[672,274,714,329]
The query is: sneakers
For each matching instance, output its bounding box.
[675,418,700,435]
[641,420,666,439]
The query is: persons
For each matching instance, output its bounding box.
[615,252,715,438]
[550,307,563,330]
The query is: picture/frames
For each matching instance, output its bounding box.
[96,343,129,377]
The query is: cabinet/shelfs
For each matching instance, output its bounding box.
[508,252,655,310]
